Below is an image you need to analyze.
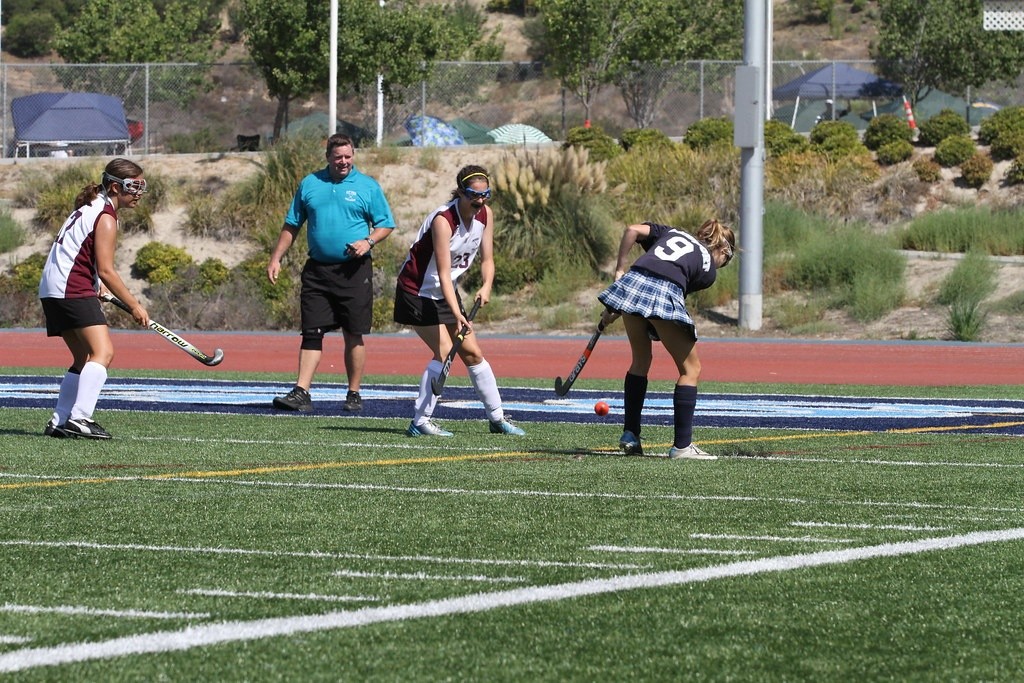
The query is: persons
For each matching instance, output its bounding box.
[267,134,394,411]
[600,221,735,460]
[34,145,81,159]
[39,158,152,440]
[818,99,852,123]
[392,166,528,437]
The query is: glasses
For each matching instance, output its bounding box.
[721,239,733,267]
[105,172,148,196]
[460,181,491,200]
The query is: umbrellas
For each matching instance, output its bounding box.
[485,124,552,144]
[407,113,467,146]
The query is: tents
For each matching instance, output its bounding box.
[387,117,494,147]
[278,111,374,142]
[774,65,999,134]
[12,93,131,144]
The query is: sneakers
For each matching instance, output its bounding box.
[670,442,718,459]
[273,387,313,411]
[343,391,361,412]
[63,415,112,440]
[618,430,643,456]
[407,419,453,437]
[44,420,78,439]
[489,415,525,435]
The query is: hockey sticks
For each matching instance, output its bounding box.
[432,297,482,396]
[555,307,613,396]
[99,293,223,367]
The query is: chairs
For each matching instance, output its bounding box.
[237,133,260,151]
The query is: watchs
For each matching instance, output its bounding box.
[365,237,374,249]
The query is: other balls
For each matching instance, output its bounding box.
[594,402,609,416]
